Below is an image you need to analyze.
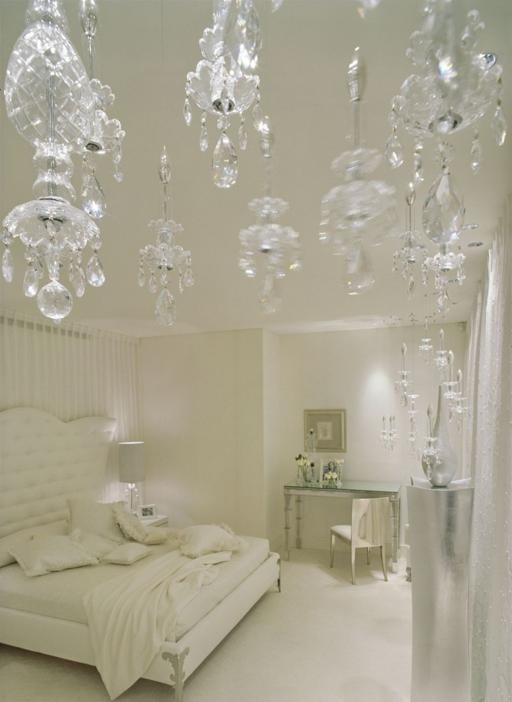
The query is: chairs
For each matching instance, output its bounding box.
[329,496,389,585]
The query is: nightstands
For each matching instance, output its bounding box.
[141,515,169,528]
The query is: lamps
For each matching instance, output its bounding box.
[136,147,196,323]
[387,0,507,312]
[185,0,278,186]
[236,197,304,313]
[117,441,147,514]
[1,2,127,320]
[320,0,397,295]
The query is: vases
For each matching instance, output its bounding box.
[422,385,458,486]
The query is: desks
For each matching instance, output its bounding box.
[283,480,401,564]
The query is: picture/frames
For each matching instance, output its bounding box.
[138,504,157,518]
[304,407,348,455]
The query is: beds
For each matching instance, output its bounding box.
[1,408,282,702]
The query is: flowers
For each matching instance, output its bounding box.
[296,454,340,488]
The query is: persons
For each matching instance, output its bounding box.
[323,459,338,486]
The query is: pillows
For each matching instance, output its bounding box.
[177,524,242,558]
[11,500,168,577]
